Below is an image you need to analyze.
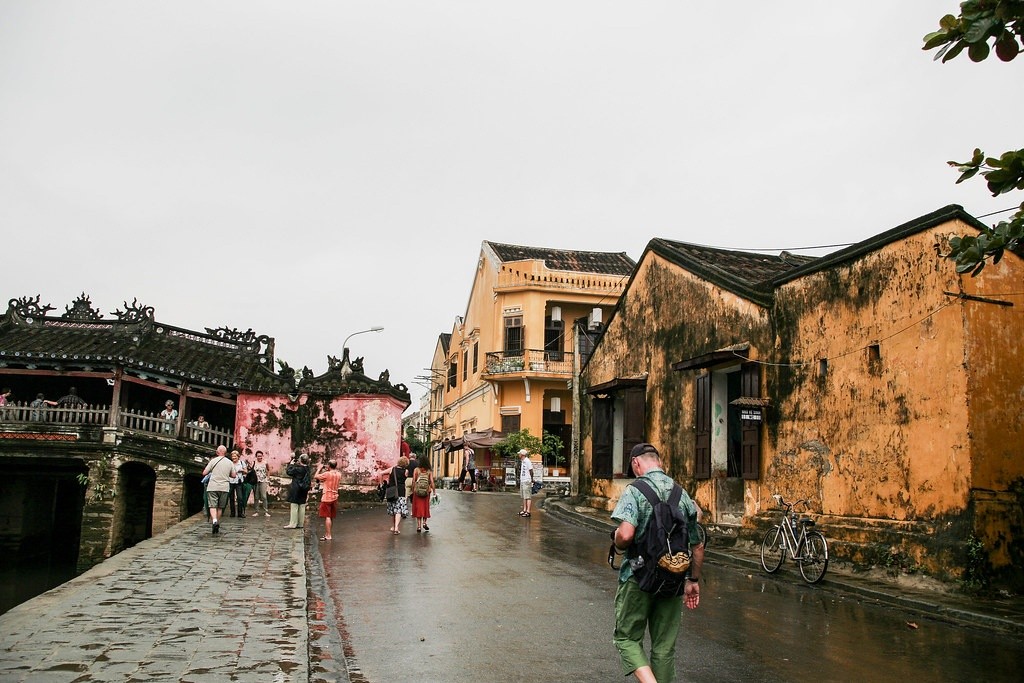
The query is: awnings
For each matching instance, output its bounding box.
[432,438,450,451]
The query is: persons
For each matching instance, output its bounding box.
[313,459,341,540]
[455,443,477,492]
[517,449,533,517]
[161,400,178,435]
[30,393,50,421]
[201,444,270,534]
[610,443,704,683]
[0,387,11,407]
[385,434,436,535]
[43,387,85,423]
[283,454,312,528]
[187,415,209,441]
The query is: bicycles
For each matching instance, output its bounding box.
[760,494,829,584]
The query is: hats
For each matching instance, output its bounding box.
[627,443,659,478]
[517,449,527,456]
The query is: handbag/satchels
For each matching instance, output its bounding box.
[246,468,258,486]
[463,457,475,470]
[386,485,399,502]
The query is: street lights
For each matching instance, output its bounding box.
[342,327,385,360]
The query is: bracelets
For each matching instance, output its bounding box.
[685,577,699,582]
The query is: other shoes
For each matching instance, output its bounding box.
[238,514,246,518]
[457,488,462,491]
[394,530,400,535]
[297,524,304,528]
[212,521,220,534]
[320,535,327,541]
[522,512,531,517]
[471,488,477,492]
[252,512,260,517]
[230,513,236,517]
[517,510,526,515]
[283,525,296,529]
[417,527,421,533]
[422,523,429,530]
[264,513,271,516]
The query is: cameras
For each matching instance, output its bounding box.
[610,528,617,541]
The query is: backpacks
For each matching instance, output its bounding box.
[628,477,692,597]
[412,467,432,498]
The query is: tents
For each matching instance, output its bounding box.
[445,427,519,454]
